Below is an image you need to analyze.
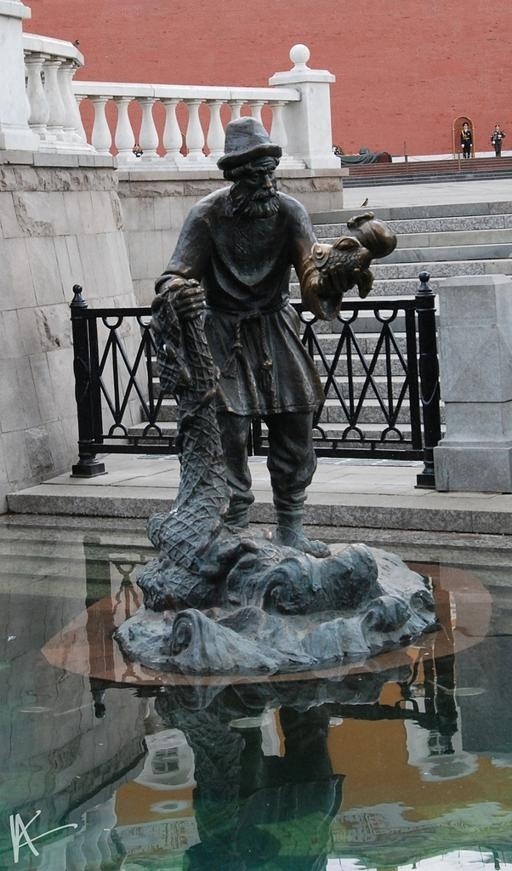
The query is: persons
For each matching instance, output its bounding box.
[460,122,473,158]
[133,144,143,158]
[154,114,396,558]
[490,124,506,157]
[183,709,346,871]
